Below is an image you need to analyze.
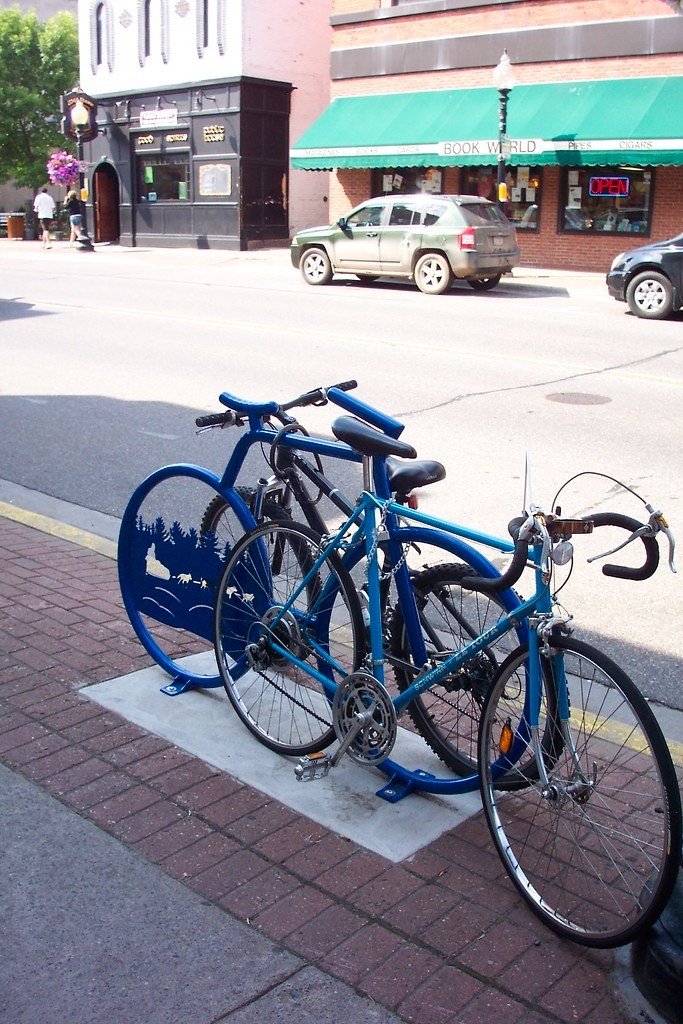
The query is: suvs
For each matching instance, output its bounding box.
[607,231,683,321]
[291,194,521,296]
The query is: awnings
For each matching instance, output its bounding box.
[290,75,682,170]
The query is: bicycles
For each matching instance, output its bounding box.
[118,389,541,805]
[194,380,571,791]
[214,414,683,948]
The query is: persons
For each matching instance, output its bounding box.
[64,190,83,248]
[33,188,56,249]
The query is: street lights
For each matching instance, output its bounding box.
[492,48,515,214]
[71,101,95,251]
